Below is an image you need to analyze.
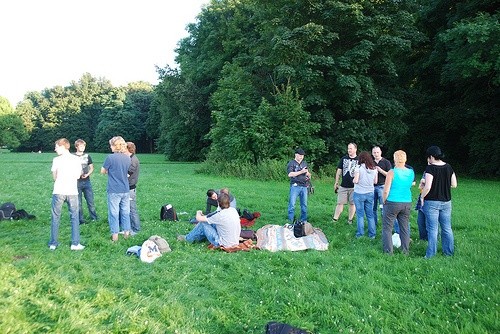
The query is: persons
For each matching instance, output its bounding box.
[287,149,311,229]
[415,155,432,241]
[352,150,378,239]
[48,138,86,251]
[204,189,221,215]
[177,197,241,248]
[331,143,361,225]
[381,149,415,258]
[391,164,417,234]
[190,188,237,224]
[371,146,391,226]
[101,136,132,241]
[123,142,143,232]
[73,139,100,223]
[421,146,458,259]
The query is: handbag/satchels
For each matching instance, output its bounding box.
[294,220,304,238]
[302,220,315,235]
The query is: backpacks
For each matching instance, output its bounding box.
[239,230,257,242]
[0,202,16,221]
[140,239,162,263]
[148,234,171,253]
[244,209,254,220]
[125,245,141,257]
[236,208,241,216]
[14,209,36,220]
[161,204,179,221]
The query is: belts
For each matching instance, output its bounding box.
[374,184,384,186]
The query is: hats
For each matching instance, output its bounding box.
[295,149,305,155]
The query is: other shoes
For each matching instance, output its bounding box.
[331,219,337,222]
[348,220,353,225]
[284,222,292,228]
[119,231,130,236]
[50,246,56,250]
[112,234,119,241]
[176,232,186,241]
[71,243,85,251]
